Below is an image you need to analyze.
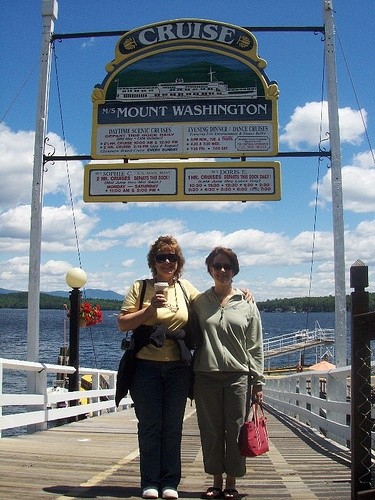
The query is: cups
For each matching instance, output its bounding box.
[154,281,169,303]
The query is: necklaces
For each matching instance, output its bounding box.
[166,280,179,313]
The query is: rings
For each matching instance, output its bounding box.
[260,398,262,400]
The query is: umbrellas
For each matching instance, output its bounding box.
[308,361,336,369]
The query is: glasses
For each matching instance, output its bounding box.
[211,264,232,271]
[155,254,178,263]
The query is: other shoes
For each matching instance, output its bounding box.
[142,486,159,498]
[162,486,179,499]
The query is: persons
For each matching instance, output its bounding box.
[191,246,266,500]
[115,236,254,499]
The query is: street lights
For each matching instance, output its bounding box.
[66,267,87,423]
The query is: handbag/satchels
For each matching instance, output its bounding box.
[238,399,270,457]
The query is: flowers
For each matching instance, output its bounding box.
[63,302,103,326]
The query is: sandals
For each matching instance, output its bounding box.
[223,488,239,500]
[201,487,221,498]
